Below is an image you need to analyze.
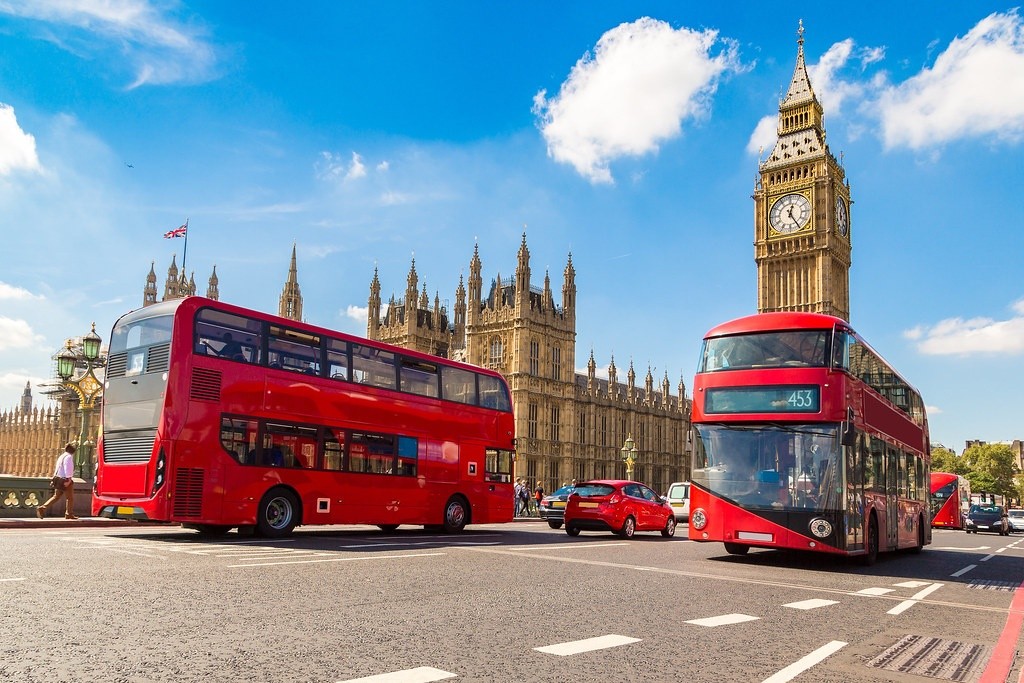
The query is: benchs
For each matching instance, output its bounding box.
[247,448,285,467]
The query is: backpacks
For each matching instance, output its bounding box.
[535,490,541,500]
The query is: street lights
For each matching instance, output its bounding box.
[58,321,109,478]
[621,432,638,481]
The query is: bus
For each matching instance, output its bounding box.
[90,298,519,540]
[689,312,931,568]
[930,472,972,530]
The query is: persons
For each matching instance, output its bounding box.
[36,443,80,519]
[730,461,759,496]
[931,500,946,514]
[203,332,248,362]
[974,506,984,513]
[660,492,667,500]
[535,481,544,518]
[561,483,566,488]
[571,479,576,486]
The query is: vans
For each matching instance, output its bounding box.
[666,482,690,523]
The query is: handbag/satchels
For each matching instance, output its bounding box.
[52,476,67,490]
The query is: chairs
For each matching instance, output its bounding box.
[232,354,248,363]
[358,379,373,384]
[301,367,317,375]
[331,373,346,379]
[268,360,283,367]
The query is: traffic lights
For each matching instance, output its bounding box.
[990,494,994,505]
[980,490,986,502]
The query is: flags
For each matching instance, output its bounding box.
[163,222,187,239]
[514,477,533,518]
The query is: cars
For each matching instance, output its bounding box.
[1008,509,1024,533]
[966,504,1010,536]
[539,484,574,529]
[564,480,676,540]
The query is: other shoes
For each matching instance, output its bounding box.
[65,512,79,519]
[36,505,45,518]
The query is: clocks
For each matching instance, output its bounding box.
[836,195,848,237]
[769,193,811,233]
